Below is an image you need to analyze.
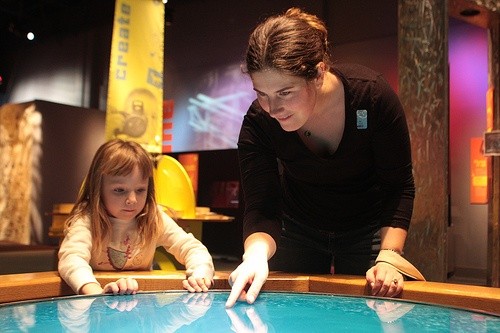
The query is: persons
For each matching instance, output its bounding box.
[226,299,415,333]
[226,8,416,308]
[57,292,214,333]
[57,138,215,295]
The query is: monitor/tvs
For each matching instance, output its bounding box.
[162,149,244,211]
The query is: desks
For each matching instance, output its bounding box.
[0,271,500,333]
[169,209,234,223]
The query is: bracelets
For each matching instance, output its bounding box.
[384,248,402,255]
[383,319,399,324]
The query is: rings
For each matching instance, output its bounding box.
[392,278,398,284]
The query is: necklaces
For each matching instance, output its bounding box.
[303,125,312,136]
